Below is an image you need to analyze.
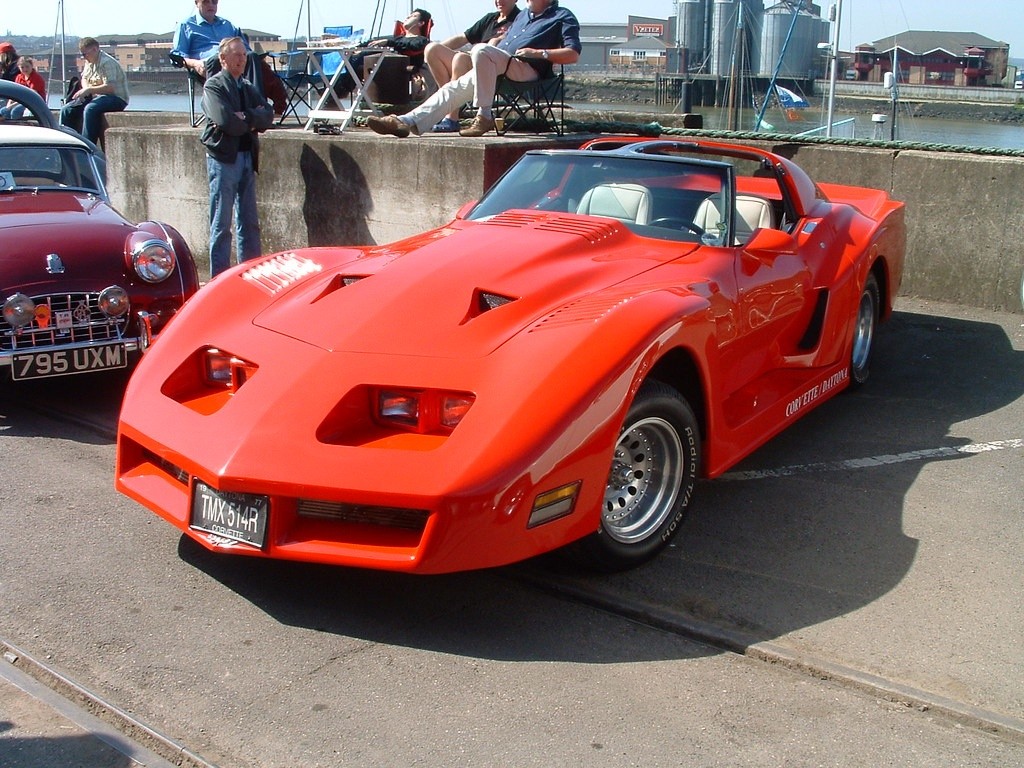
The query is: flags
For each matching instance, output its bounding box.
[751,85,810,132]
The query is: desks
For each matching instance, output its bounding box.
[297,46,394,134]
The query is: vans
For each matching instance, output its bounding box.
[1014,81,1023,90]
[845,70,857,81]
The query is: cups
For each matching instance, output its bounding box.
[494,118,504,131]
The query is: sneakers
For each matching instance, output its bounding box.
[459,116,494,137]
[367,113,409,137]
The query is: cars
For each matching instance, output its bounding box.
[0,79,106,196]
[0,124,204,416]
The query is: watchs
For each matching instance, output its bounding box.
[541,48,548,60]
[242,111,246,120]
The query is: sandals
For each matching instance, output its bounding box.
[432,116,460,132]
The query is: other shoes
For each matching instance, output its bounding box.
[320,99,339,111]
[317,87,349,100]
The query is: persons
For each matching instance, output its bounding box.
[315,0,583,137]
[199,36,274,281]
[170,0,276,129]
[0,37,129,162]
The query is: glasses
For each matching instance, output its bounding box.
[201,0,218,5]
[80,48,94,58]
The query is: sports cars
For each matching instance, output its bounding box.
[114,135,909,576]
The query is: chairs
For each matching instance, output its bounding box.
[687,193,773,246]
[351,19,434,121]
[170,34,275,128]
[270,26,353,128]
[574,181,652,227]
[437,39,564,141]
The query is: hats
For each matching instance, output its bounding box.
[0,42,14,53]
